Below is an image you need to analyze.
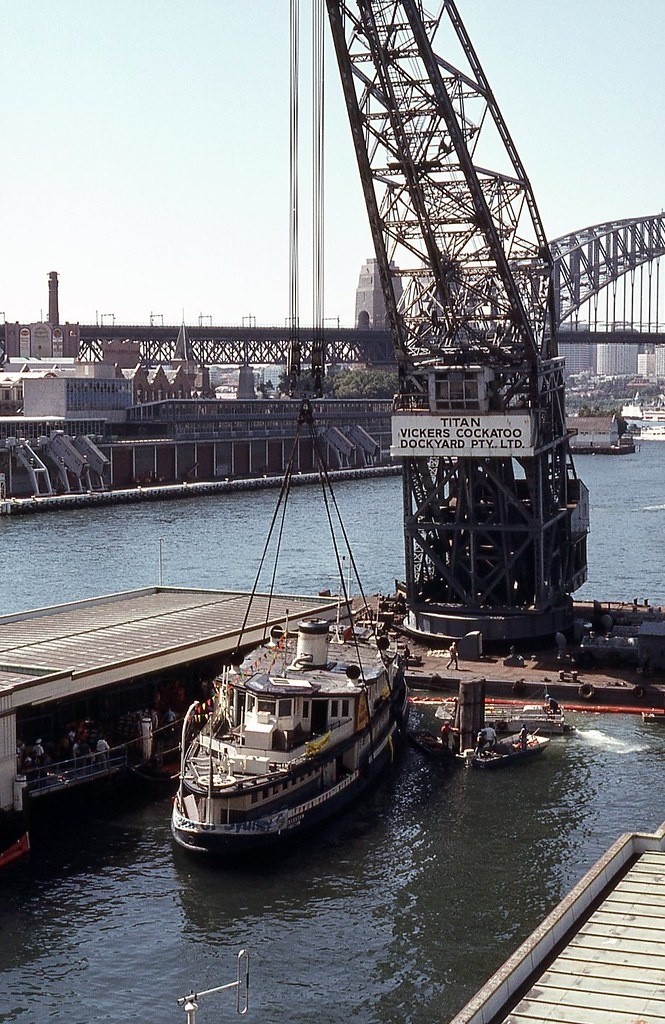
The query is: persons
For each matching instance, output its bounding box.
[403,644,410,670]
[446,642,458,670]
[545,694,558,714]
[474,722,497,759]
[519,723,528,749]
[441,722,460,748]
[16,675,210,783]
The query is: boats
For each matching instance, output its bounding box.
[455,733,550,769]
[434,701,576,734]
[172,615,412,870]
[407,729,443,756]
[622,425,665,441]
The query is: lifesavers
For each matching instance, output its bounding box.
[632,685,645,699]
[578,683,595,700]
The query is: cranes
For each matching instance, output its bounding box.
[324,0,593,620]
